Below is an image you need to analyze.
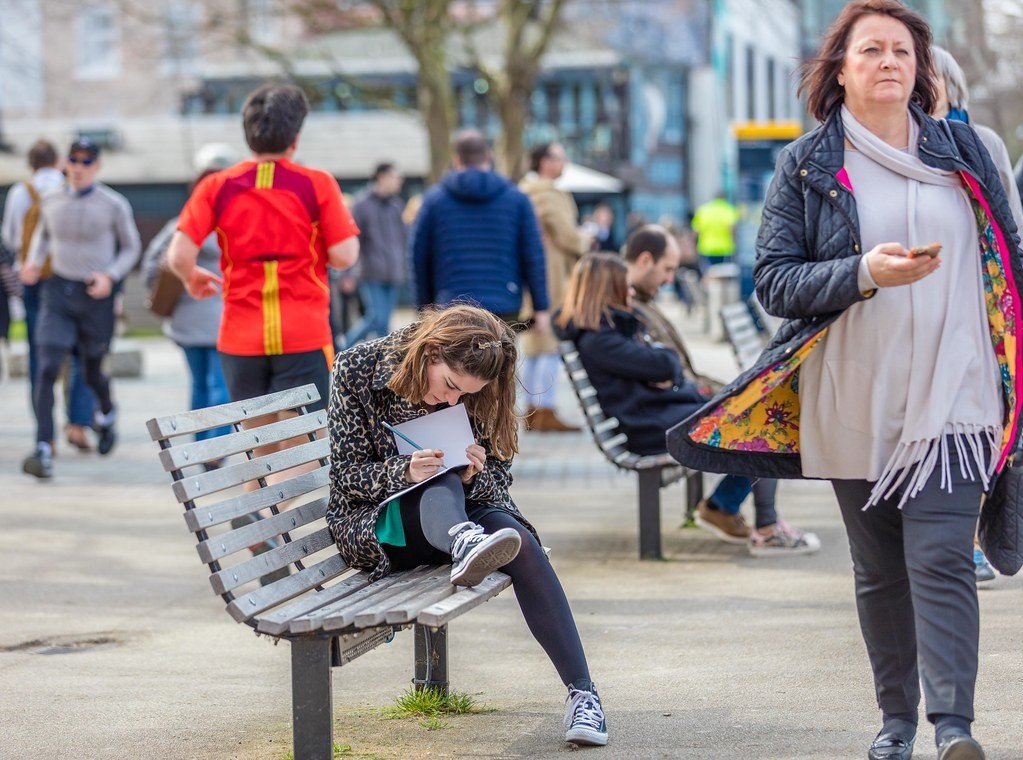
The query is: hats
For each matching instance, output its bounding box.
[70,140,99,155]
[68,154,95,165]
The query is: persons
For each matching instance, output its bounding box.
[0,136,231,479]
[331,128,596,421]
[573,184,746,306]
[167,82,359,587]
[665,2,1023,760]
[326,294,606,745]
[917,46,1023,250]
[552,224,821,558]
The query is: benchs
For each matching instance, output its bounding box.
[675,266,706,316]
[749,289,784,338]
[718,300,763,373]
[551,332,706,558]
[146,382,556,760]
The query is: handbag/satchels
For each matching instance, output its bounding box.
[148,254,182,320]
[977,447,1023,576]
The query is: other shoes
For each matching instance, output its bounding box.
[525,406,581,431]
[21,452,52,478]
[60,421,92,451]
[98,422,114,454]
[972,549,996,583]
[230,512,292,586]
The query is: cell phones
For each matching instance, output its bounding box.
[907,244,942,260]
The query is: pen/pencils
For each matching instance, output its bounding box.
[381,421,449,470]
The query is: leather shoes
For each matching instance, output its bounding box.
[868,722,917,760]
[937,734,986,760]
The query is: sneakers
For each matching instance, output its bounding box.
[747,519,821,555]
[692,498,753,544]
[563,681,609,746]
[448,520,523,587]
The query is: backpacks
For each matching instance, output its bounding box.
[20,177,54,279]
